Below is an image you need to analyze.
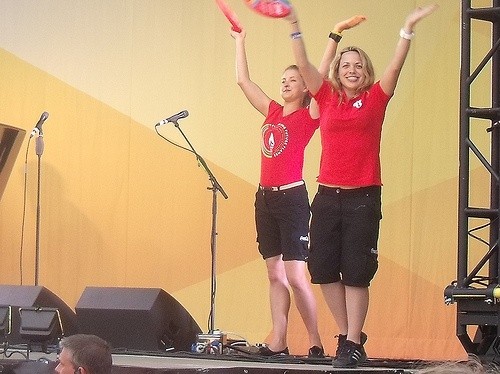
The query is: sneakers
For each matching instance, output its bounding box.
[255,342,290,357]
[308,346,324,359]
[332,331,368,367]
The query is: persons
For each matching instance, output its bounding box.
[232,17,367,359]
[53,334,111,374]
[281,4,438,367]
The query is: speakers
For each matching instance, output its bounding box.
[74,287,204,350]
[0,285,76,351]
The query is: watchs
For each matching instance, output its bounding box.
[400,27,415,42]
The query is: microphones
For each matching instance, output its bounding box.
[157,111,188,126]
[30,111,49,138]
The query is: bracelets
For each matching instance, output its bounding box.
[290,33,302,39]
[289,20,298,24]
[328,31,342,42]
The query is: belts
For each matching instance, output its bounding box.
[260,181,304,192]
